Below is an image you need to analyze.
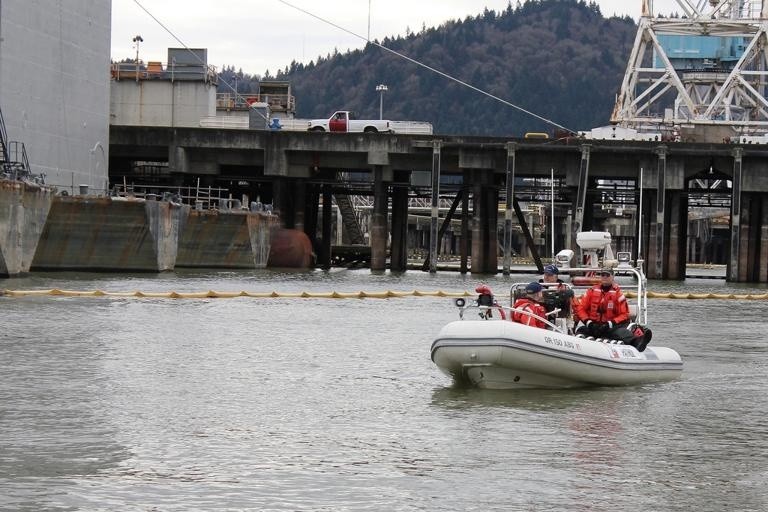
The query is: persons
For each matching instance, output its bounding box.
[509,281,546,329]
[537,265,579,336]
[575,266,652,352]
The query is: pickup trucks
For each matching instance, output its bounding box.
[307,108,392,137]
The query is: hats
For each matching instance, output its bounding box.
[543,264,558,276]
[524,281,543,295]
[596,266,614,276]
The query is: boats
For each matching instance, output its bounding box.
[426,168,681,390]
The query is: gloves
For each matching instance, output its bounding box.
[586,319,613,337]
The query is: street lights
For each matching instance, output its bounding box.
[232,73,240,114]
[132,34,143,83]
[376,82,388,119]
